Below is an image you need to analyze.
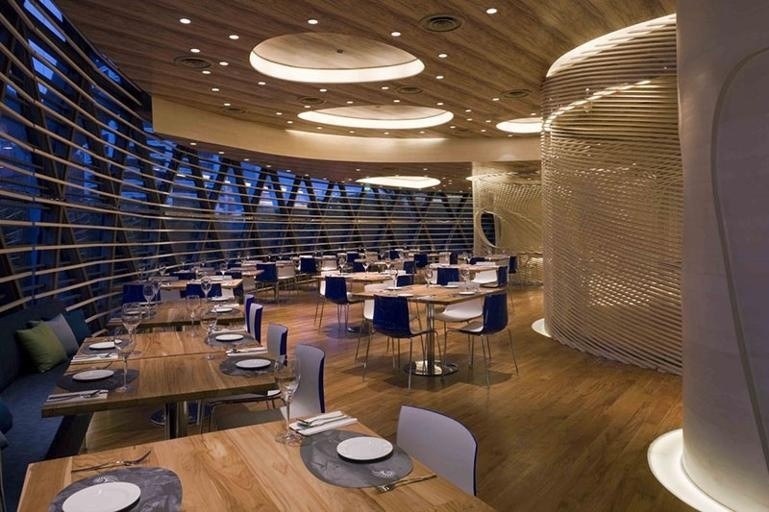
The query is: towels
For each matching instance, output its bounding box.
[43,389,109,405]
[71,353,119,362]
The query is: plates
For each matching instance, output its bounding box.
[335,434,394,463]
[60,480,142,512]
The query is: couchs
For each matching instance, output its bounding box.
[0,298,93,512]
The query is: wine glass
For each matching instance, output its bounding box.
[272,358,303,444]
[46,249,272,400]
[277,241,506,296]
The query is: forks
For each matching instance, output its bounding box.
[372,474,436,494]
[69,450,152,473]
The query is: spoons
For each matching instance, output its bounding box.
[295,413,345,426]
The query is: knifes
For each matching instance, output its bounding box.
[293,416,349,432]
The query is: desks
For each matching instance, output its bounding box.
[17,410,498,512]
[69,325,264,364]
[41,347,295,440]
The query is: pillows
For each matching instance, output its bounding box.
[28,313,79,355]
[64,309,92,344]
[0,430,9,450]
[0,402,13,433]
[16,320,68,373]
